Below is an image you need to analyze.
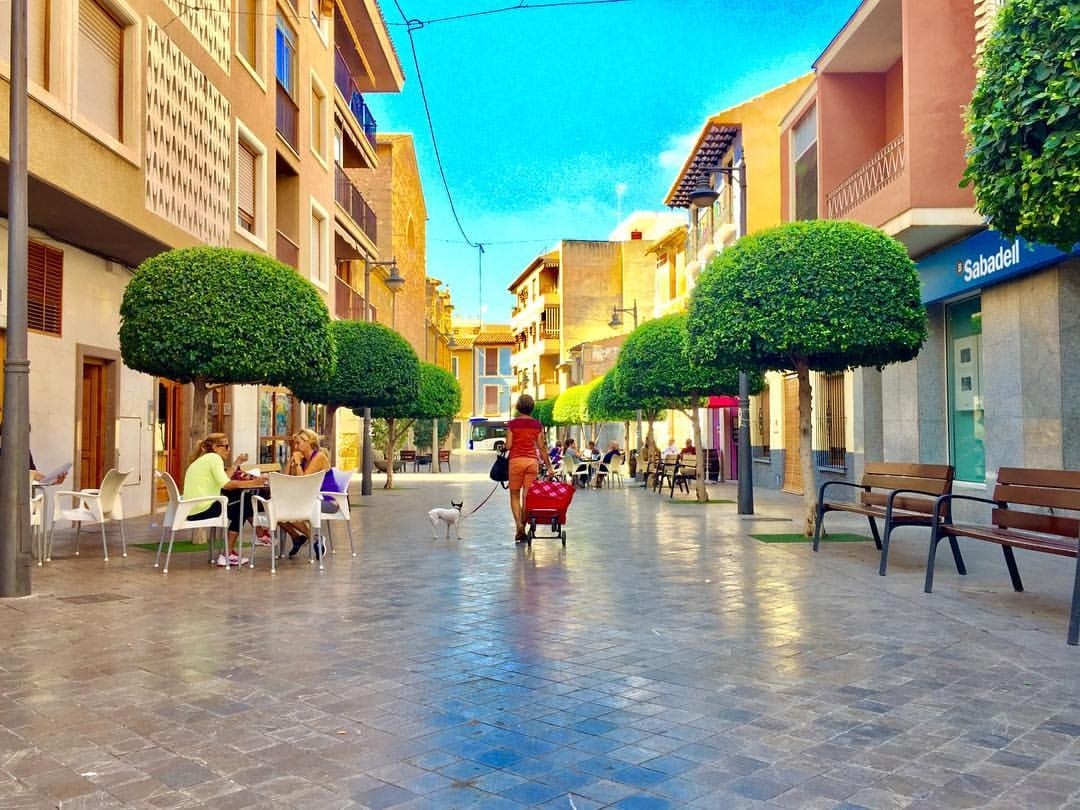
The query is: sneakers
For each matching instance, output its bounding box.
[314,535,327,561]
[288,535,309,559]
[216,550,249,565]
[255,533,278,546]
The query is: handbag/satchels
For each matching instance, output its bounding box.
[231,466,258,480]
[525,476,576,508]
[490,446,509,481]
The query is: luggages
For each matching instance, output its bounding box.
[525,474,575,546]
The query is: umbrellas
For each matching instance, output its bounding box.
[702,395,739,484]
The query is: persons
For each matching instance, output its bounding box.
[258,428,341,561]
[549,439,697,488]
[0,421,70,487]
[183,432,272,566]
[502,394,555,542]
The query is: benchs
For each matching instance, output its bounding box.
[811,461,966,576]
[926,467,1080,645]
[645,454,698,497]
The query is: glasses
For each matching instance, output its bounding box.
[217,445,229,451]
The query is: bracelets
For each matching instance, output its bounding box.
[295,464,303,466]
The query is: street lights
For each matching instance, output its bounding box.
[362,253,406,495]
[689,146,754,514]
[607,298,643,482]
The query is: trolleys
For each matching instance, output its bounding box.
[523,475,574,546]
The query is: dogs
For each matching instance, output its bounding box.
[428,500,464,540]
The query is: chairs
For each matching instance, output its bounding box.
[28,468,357,572]
[398,450,451,473]
[549,453,624,489]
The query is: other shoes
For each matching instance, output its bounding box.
[515,527,527,539]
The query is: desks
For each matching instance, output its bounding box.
[578,462,598,486]
[221,482,269,568]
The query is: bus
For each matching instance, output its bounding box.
[468,417,513,451]
